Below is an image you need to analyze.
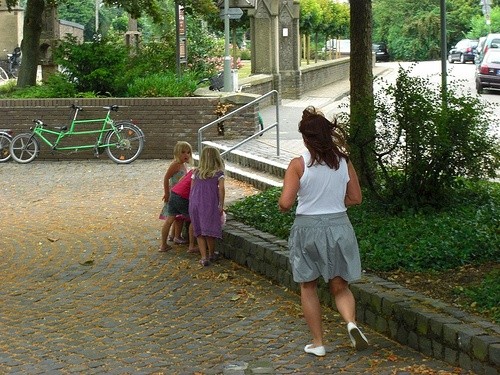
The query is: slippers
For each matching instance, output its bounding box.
[159,245,172,252]
[187,248,197,252]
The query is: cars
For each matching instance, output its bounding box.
[372,43,390,61]
[475,48,500,94]
[474,37,487,62]
[448,39,479,64]
[478,34,500,65]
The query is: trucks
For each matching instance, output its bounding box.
[321,38,351,56]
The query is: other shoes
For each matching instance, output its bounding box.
[347,322,368,351]
[173,237,186,243]
[199,254,220,266]
[304,344,325,356]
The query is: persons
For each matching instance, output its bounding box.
[279,106,368,356]
[159,141,192,244]
[158,169,200,254]
[189,146,225,267]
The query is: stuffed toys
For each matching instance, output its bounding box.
[221,211,226,224]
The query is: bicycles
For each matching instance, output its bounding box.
[197,71,224,92]
[8,104,146,164]
[0,49,22,81]
[0,129,14,162]
[234,82,264,137]
